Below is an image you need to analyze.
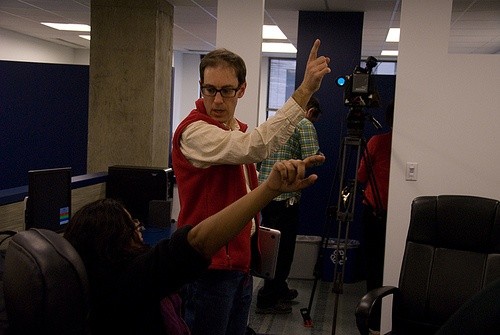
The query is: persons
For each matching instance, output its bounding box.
[0,155,326,335]
[255,97,324,314]
[358,102,394,293]
[172,39,331,335]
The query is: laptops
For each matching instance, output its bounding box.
[252,224,282,280]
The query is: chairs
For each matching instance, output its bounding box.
[355,194,500,335]
[0,226,90,335]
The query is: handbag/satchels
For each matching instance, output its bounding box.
[341,181,362,209]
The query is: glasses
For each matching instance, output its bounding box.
[200,82,243,98]
[130,219,144,232]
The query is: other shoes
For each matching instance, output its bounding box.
[281,289,298,300]
[255,303,292,313]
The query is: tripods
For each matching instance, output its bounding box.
[298,116,385,335]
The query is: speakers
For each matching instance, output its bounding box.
[26,167,71,234]
[107,165,174,228]
